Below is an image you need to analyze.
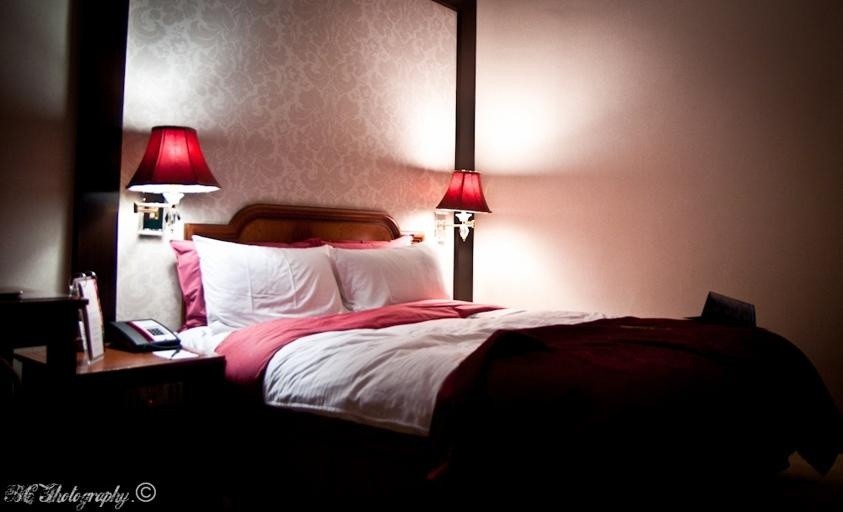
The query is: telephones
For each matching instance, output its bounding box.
[111,318,181,353]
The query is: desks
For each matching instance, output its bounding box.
[0,285,86,416]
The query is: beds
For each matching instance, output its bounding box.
[169,204,841,511]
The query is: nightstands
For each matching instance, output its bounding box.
[12,345,229,510]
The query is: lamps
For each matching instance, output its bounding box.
[125,126,221,236]
[436,169,493,244]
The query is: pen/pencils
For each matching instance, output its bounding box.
[172,350,179,357]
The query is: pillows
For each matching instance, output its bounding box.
[327,235,419,251]
[169,237,325,329]
[194,232,343,330]
[326,240,450,313]
